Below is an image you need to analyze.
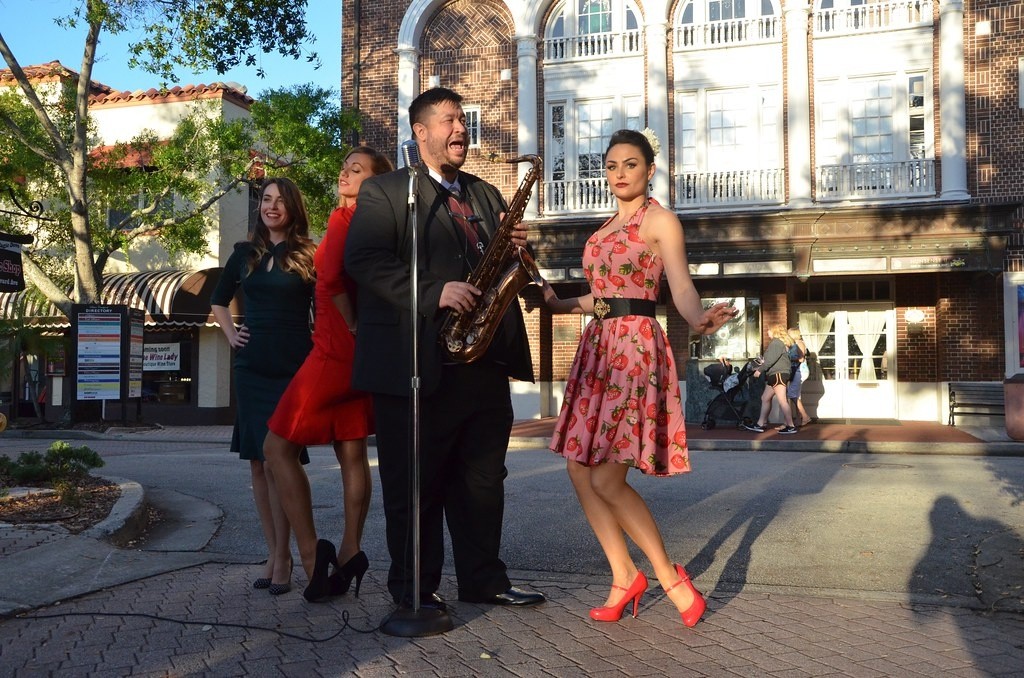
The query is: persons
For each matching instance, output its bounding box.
[209,177,318,595]
[743,321,798,434]
[775,327,812,433]
[540,129,736,627]
[341,87,545,610]
[263,146,394,601]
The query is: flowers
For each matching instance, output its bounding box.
[640,127,661,157]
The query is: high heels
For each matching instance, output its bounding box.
[253,556,293,595]
[664,563,706,628]
[590,571,648,622]
[303,539,369,602]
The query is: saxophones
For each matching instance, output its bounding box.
[434,150,545,366]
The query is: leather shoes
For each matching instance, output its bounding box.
[478,586,547,608]
[393,592,447,611]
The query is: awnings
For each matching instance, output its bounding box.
[0,267,246,332]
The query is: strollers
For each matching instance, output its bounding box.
[701,356,758,431]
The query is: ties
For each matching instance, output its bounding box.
[452,185,461,197]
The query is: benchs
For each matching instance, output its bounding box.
[947,382,1006,427]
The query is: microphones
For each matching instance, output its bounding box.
[402,140,422,168]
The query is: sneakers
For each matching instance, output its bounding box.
[777,425,797,434]
[745,422,764,433]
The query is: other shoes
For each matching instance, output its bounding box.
[794,417,812,426]
[774,423,786,430]
